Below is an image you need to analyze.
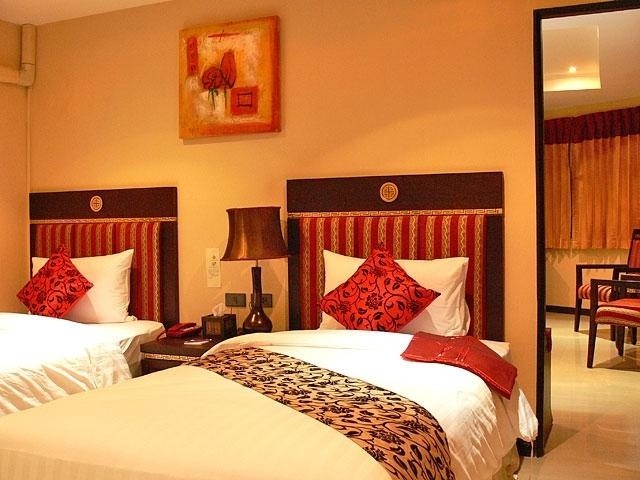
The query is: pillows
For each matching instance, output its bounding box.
[16,245,141,324]
[315,238,476,338]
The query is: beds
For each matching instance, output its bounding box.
[0,185,182,420]
[0,169,539,480]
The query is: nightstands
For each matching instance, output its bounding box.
[138,332,222,373]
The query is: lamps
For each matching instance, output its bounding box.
[217,205,292,332]
[541,24,602,94]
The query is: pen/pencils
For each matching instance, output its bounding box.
[190,339,212,342]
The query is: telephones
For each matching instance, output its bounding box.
[166,322,202,338]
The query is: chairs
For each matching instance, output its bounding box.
[574,227,640,369]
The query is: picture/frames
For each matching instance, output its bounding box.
[178,15,282,139]
